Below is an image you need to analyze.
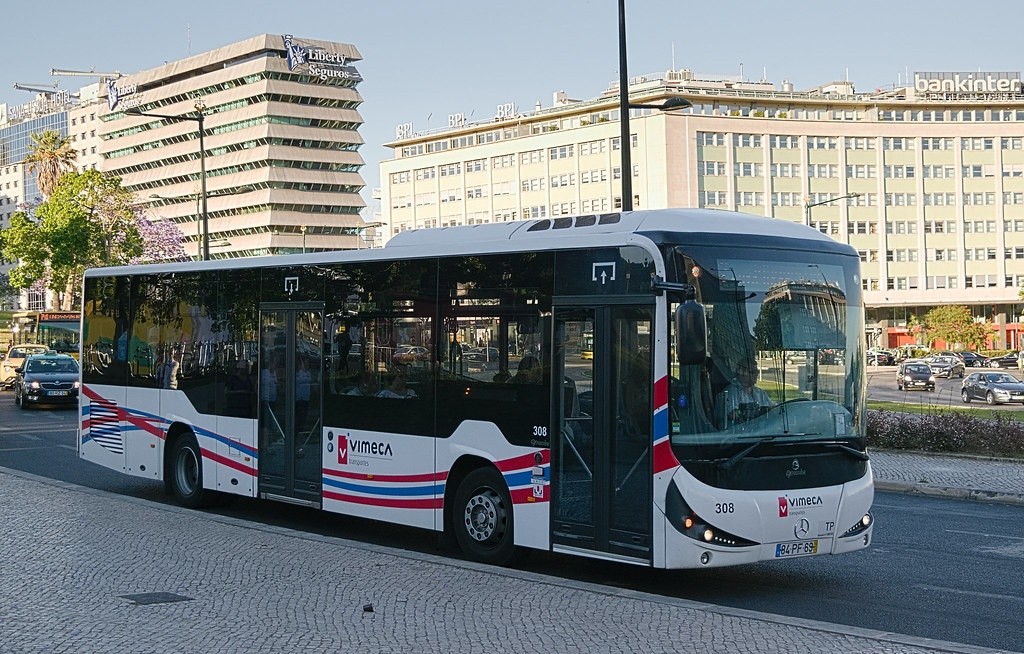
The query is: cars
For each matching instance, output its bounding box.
[0,344,50,390]
[785,342,989,373]
[929,356,966,379]
[323,336,501,364]
[13,349,81,409]
[896,362,935,392]
[982,350,1021,368]
[961,372,1024,405]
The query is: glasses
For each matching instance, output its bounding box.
[743,370,760,375]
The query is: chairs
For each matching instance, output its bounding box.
[31,361,41,371]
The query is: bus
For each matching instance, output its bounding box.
[81,207,876,568]
[12,310,81,362]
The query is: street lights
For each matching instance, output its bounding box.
[123,96,209,260]
[148,187,255,260]
[803,192,861,225]
[617,1,693,212]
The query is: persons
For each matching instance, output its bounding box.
[714,358,778,429]
[504,357,543,385]
[158,345,312,444]
[58,364,72,372]
[349,371,420,401]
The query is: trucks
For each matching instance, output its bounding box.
[0,329,13,360]
